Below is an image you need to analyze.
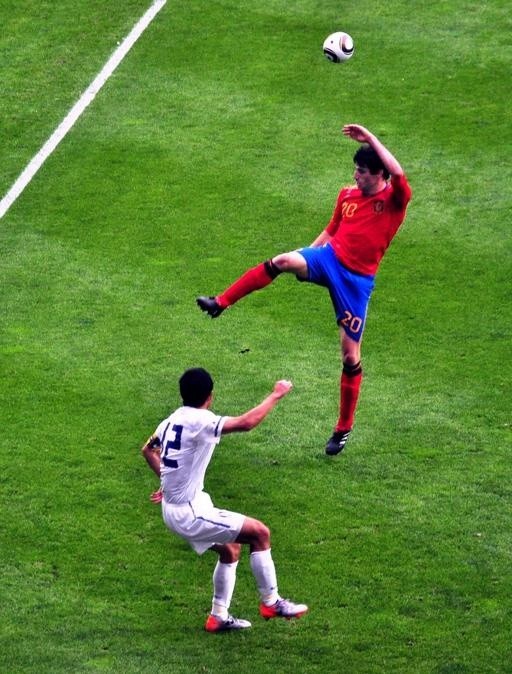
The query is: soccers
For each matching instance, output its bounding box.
[323,31,353,63]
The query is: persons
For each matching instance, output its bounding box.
[194,122,412,456]
[140,365,310,633]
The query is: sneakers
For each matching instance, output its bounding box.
[259,596,308,620]
[196,296,225,318]
[326,427,351,455]
[205,612,252,632]
